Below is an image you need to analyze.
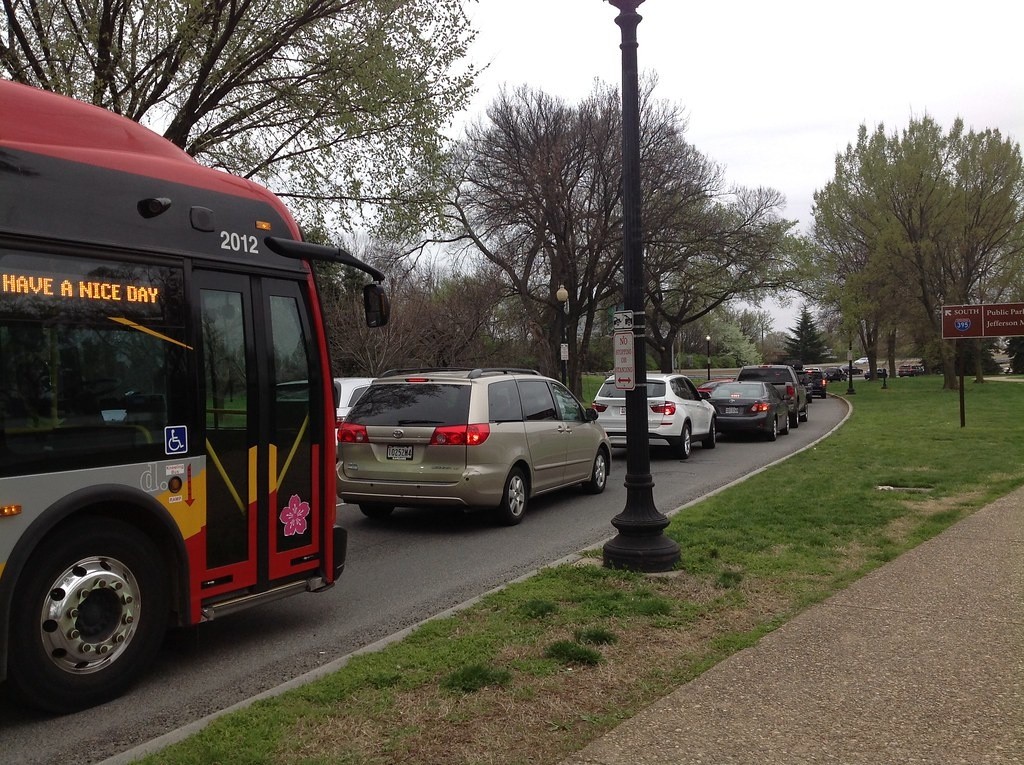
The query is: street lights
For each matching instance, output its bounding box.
[556,282,569,387]
[705,334,712,382]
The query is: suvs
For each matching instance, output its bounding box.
[337,364,613,528]
[277,376,382,465]
[591,371,720,458]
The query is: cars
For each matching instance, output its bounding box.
[698,351,928,442]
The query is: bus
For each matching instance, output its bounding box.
[1,72,392,722]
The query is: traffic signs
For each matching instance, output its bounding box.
[941,302,1024,340]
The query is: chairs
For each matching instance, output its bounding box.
[719,389,731,397]
[490,395,510,420]
[747,388,760,397]
